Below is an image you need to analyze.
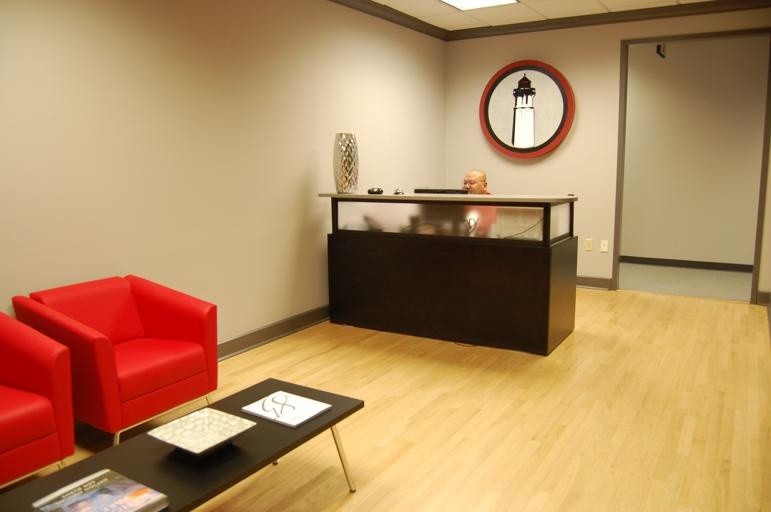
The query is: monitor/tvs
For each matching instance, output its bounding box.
[414,189,468,224]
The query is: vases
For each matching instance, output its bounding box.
[332,133,359,196]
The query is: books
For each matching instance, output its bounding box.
[32,466,171,511]
[240,389,333,429]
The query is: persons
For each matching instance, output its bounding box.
[463,169,497,236]
